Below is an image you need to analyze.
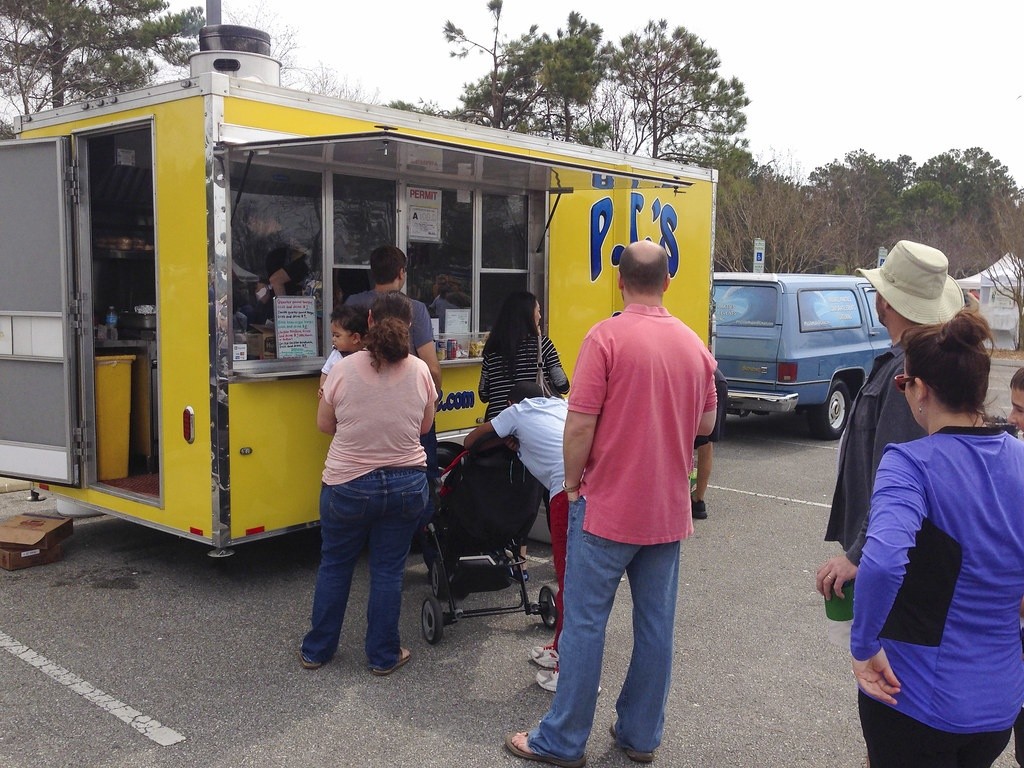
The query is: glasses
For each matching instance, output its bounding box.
[895,374,916,393]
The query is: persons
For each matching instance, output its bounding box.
[231,200,308,332]
[506,239,718,768]
[299,293,439,673]
[430,219,506,329]
[818,240,965,601]
[612,312,728,519]
[463,382,601,696]
[1007,368,1024,768]
[850,305,1024,768]
[478,291,571,582]
[343,247,442,570]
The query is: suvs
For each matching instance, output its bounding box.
[713,269,892,441]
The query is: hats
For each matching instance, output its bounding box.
[854,239,965,325]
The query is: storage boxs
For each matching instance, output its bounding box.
[0,544,65,570]
[0,513,74,548]
[245,323,277,356]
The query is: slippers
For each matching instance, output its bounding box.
[612,720,654,762]
[506,731,586,768]
[372,648,411,676]
[298,650,319,669]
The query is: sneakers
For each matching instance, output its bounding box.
[536,669,601,694]
[531,645,560,669]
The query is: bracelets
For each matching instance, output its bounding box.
[563,480,579,492]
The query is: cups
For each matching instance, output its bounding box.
[823,580,855,622]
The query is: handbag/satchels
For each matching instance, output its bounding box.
[537,334,566,402]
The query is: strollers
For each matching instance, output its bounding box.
[421,438,561,643]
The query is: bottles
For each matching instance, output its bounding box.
[106,306,118,340]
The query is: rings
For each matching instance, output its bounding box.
[827,574,835,581]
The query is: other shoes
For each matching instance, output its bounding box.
[691,500,707,519]
[506,561,530,583]
